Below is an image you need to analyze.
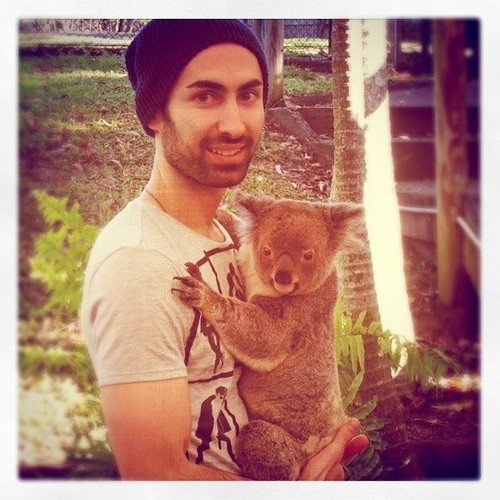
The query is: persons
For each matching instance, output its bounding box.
[81,19,369,481]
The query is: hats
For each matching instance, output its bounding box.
[123,19,269,137]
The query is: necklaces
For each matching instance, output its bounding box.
[141,184,169,214]
